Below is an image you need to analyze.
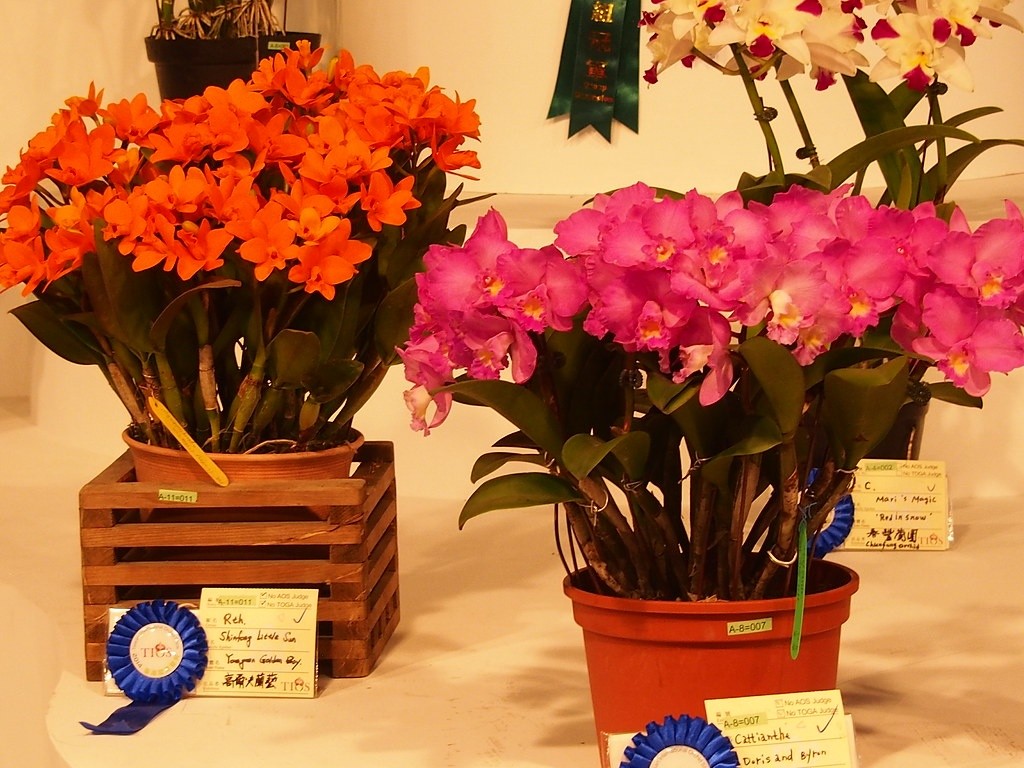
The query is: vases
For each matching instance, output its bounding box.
[142,30,326,110]
[561,555,861,768]
[77,430,401,684]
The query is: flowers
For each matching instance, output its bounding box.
[392,180,1024,582]
[639,0,1024,187]
[0,33,490,449]
